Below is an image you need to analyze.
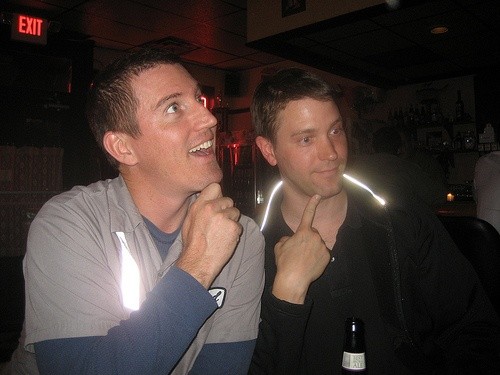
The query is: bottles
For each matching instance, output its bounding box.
[396,90,476,152]
[339,316,369,375]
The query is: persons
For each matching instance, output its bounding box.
[247,65,500,375]
[1,46,265,375]
